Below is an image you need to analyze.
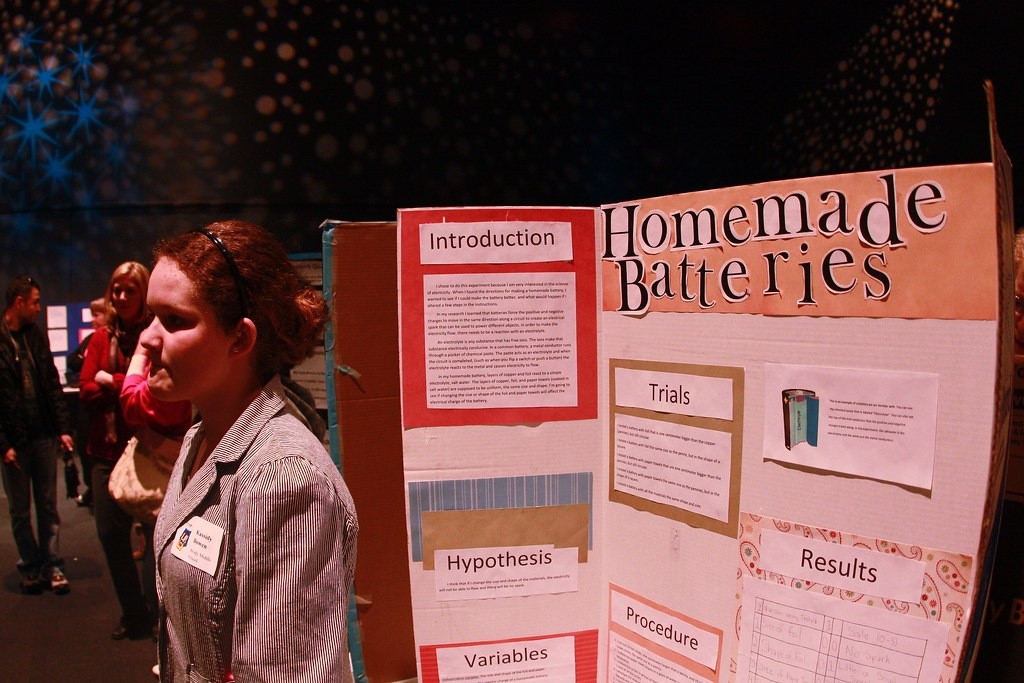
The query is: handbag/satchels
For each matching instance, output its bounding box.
[108,435,175,522]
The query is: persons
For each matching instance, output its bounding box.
[0,261,327,639]
[140,221,358,683]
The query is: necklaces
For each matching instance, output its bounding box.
[186,475,191,483]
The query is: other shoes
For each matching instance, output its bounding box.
[47,566,70,593]
[23,572,41,591]
[76,493,94,506]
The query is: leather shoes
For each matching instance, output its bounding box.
[112,622,128,640]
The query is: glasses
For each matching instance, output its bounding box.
[22,277,34,291]
